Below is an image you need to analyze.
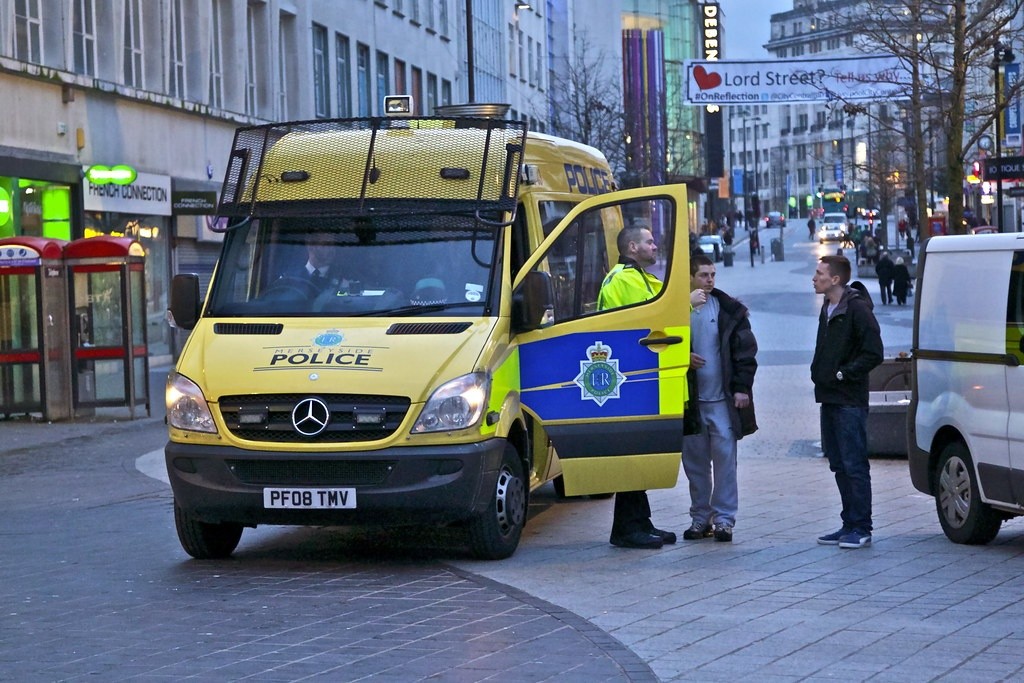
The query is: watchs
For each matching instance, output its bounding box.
[837,371,843,380]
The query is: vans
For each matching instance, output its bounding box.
[819,212,851,235]
[905,232,1023,546]
[164,93,693,560]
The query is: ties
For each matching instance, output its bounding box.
[314,270,321,279]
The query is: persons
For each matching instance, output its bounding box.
[808,217,816,240]
[298,232,338,279]
[689,229,696,246]
[843,223,881,262]
[681,254,758,543]
[719,225,733,245]
[898,219,906,239]
[810,256,884,548]
[876,251,911,305]
[701,219,716,235]
[597,225,706,550]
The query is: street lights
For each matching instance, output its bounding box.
[843,101,872,211]
[988,40,1017,233]
[752,122,772,194]
[743,117,761,232]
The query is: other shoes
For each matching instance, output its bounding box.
[882,301,886,304]
[888,299,893,303]
[642,523,677,545]
[897,302,901,305]
[610,528,663,549]
[903,301,906,304]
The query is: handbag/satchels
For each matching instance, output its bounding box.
[908,281,913,289]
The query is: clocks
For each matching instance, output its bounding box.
[979,137,991,149]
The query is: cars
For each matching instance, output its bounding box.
[699,234,727,263]
[817,223,845,244]
[765,211,787,228]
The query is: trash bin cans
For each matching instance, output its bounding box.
[770,237,785,262]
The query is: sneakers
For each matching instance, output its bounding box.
[839,532,872,548]
[817,525,852,545]
[682,520,712,540]
[715,522,733,542]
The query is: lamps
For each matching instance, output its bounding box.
[515,3,530,15]
